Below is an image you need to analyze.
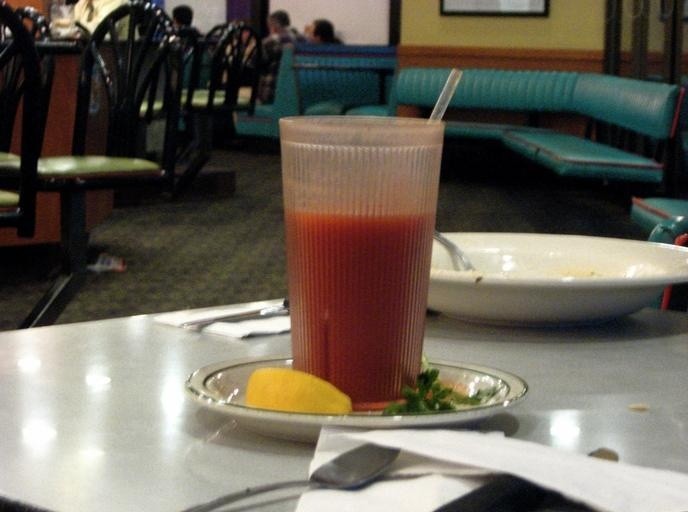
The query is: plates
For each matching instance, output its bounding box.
[187,357,526,443]
[426,230,685,329]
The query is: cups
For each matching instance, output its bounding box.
[276,113,443,399]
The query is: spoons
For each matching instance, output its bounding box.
[182,443,401,510]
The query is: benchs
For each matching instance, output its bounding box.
[234,42,400,145]
[394,63,687,252]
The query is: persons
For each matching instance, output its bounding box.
[258,10,344,105]
[50,0,78,22]
[173,5,204,38]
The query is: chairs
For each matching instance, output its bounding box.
[1,0,266,328]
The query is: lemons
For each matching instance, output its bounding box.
[245,369,354,416]
